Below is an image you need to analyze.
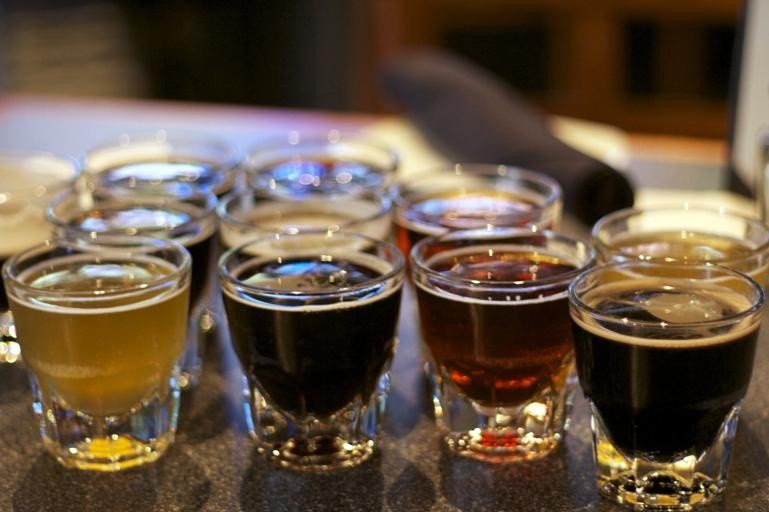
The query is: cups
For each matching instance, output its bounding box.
[217,142,408,476]
[1,138,216,476]
[389,163,598,463]
[569,205,768,512]
